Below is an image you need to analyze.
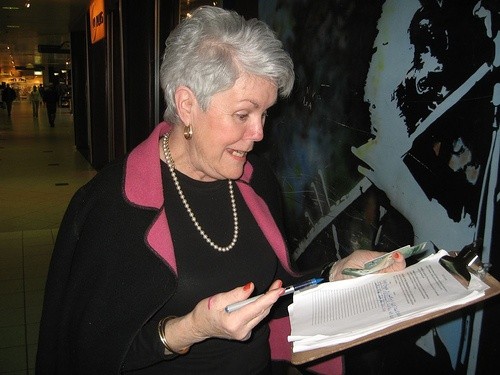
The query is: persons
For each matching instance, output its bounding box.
[34,5,406,375]
[29,86,42,120]
[38,84,45,105]
[2,83,16,118]
[42,82,60,127]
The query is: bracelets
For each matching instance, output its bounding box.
[158,316,190,355]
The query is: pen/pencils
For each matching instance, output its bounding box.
[224,277,324,314]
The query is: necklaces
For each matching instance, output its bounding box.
[163,132,238,252]
[166,129,175,168]
